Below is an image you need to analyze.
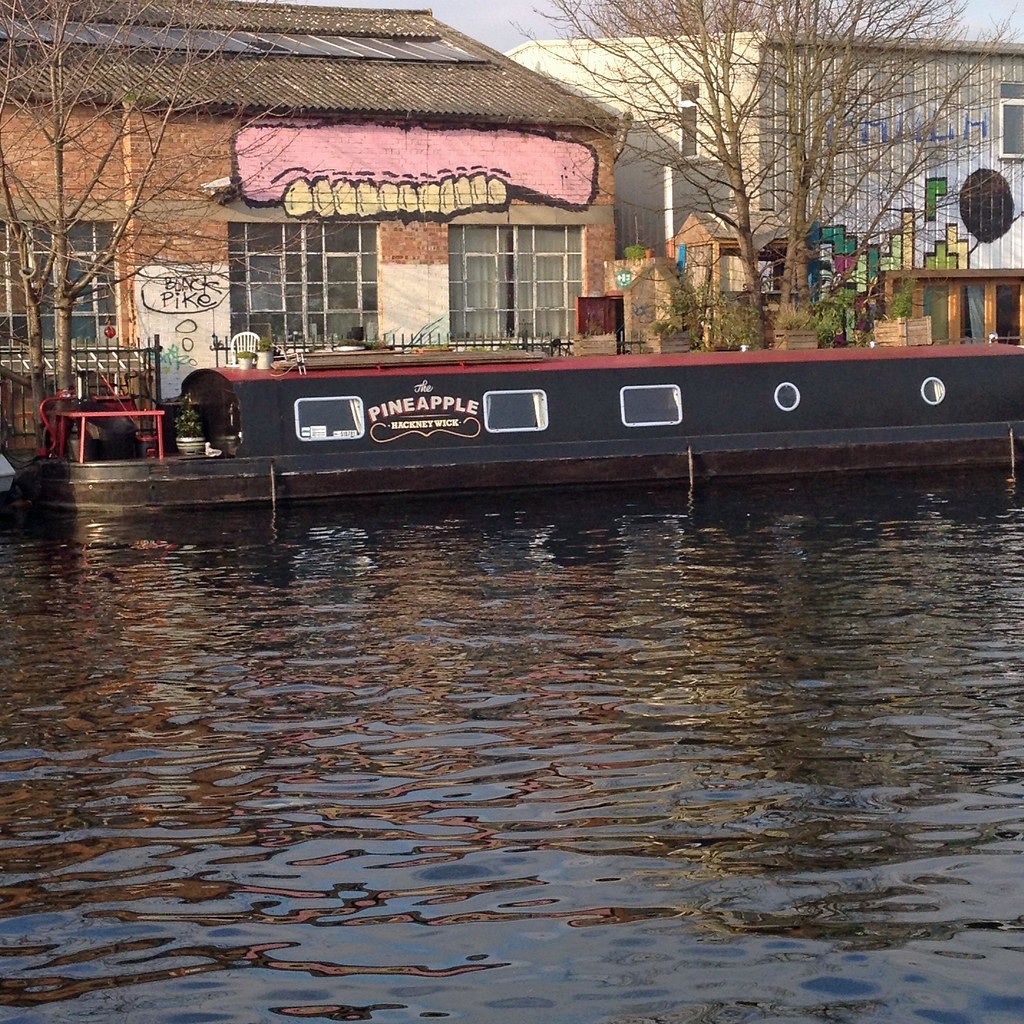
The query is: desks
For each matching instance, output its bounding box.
[56,410,165,464]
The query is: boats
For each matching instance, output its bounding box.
[16,344,1024,513]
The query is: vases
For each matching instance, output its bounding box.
[645,249,655,258]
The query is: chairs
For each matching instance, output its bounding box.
[229,332,259,366]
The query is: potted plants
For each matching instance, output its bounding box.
[647,321,690,354]
[874,285,932,347]
[235,351,256,371]
[773,310,819,350]
[256,336,272,369]
[175,409,204,457]
[573,333,617,357]
